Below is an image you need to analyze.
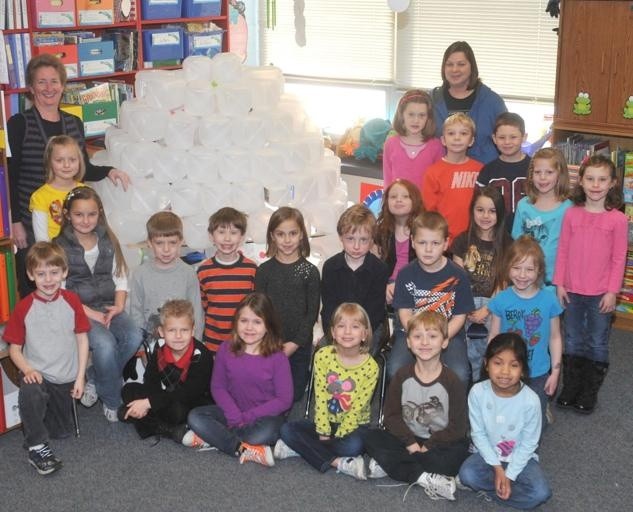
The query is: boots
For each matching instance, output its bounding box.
[575,355,609,413]
[556,354,575,407]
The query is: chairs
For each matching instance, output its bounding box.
[467,325,487,341]
[70,330,152,439]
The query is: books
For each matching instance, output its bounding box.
[60,78,133,138]
[554,132,624,166]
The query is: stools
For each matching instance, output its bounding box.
[303,342,385,428]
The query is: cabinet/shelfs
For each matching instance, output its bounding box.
[0,0,233,438]
[551,123,633,331]
[552,0,632,139]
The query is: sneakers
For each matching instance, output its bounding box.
[80,381,97,408]
[26,442,63,476]
[273,439,299,459]
[172,421,189,442]
[365,457,387,478]
[336,455,367,480]
[403,471,455,504]
[102,402,118,423]
[237,441,274,466]
[181,429,211,451]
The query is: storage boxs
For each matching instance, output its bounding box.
[184,0,222,15]
[140,0,183,21]
[31,0,76,27]
[184,30,227,58]
[75,0,114,25]
[143,28,185,59]
[78,38,116,75]
[81,101,117,136]
[34,44,79,77]
[56,104,83,122]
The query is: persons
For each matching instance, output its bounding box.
[2,242,92,476]
[551,154,629,414]
[382,89,447,189]
[5,54,133,299]
[376,113,574,368]
[29,135,90,242]
[52,187,144,423]
[429,41,509,168]
[115,202,568,510]
